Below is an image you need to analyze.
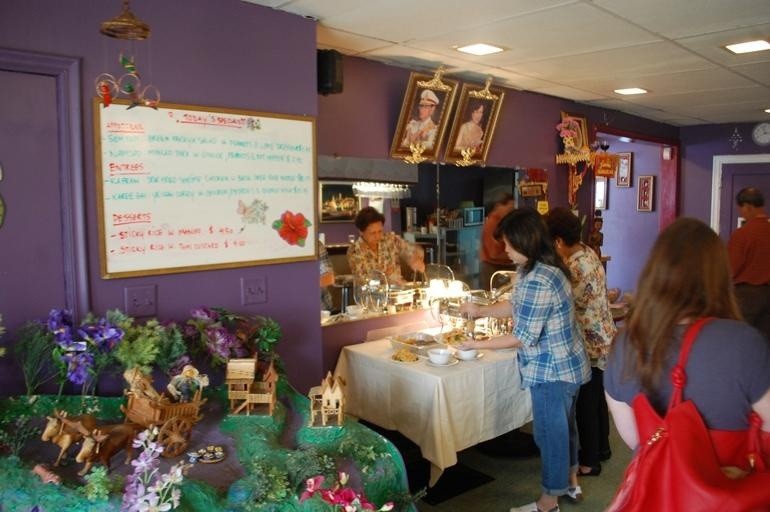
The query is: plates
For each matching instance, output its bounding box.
[387,354,425,365]
[426,358,459,366]
[433,332,464,346]
[453,352,484,361]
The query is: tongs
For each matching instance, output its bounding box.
[489,284,514,301]
[463,295,474,339]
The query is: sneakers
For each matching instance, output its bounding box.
[509,500,561,512]
[561,486,584,503]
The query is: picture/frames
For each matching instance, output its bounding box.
[318,180,362,224]
[568,115,589,150]
[386,64,459,165]
[442,76,505,167]
[595,176,608,210]
[636,176,653,212]
[615,153,631,188]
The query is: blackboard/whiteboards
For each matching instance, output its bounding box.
[94,96,318,281]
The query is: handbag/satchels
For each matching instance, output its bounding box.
[604,390,770,512]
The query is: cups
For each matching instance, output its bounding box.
[347,234,356,244]
[427,349,451,364]
[421,227,427,234]
[454,349,478,359]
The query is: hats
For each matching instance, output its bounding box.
[418,89,440,106]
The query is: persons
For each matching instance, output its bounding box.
[479,191,515,290]
[729,187,770,337]
[543,207,618,475]
[345,206,425,305]
[455,207,594,512]
[452,100,486,154]
[401,88,441,152]
[319,241,335,316]
[602,216,770,455]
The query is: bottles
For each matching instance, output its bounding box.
[412,290,420,308]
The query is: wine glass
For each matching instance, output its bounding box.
[352,182,412,199]
[590,141,610,155]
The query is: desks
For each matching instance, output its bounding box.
[600,256,611,275]
[320,304,448,383]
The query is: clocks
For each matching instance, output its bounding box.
[752,122,769,146]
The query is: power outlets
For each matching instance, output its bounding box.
[124,284,157,317]
[240,275,267,306]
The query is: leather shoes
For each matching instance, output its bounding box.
[578,448,611,460]
[573,463,602,477]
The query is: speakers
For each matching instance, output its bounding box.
[317,51,343,96]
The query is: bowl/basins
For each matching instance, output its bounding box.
[608,288,621,301]
